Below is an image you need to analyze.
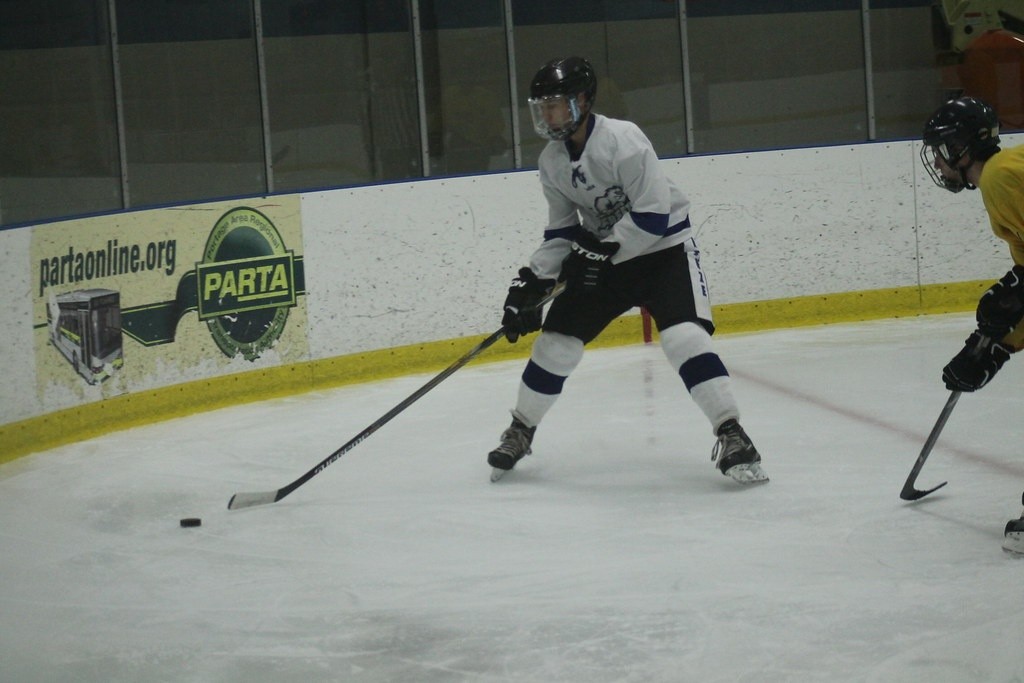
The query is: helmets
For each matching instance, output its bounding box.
[919,95,1001,194]
[527,56,599,142]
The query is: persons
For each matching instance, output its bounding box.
[485,53,769,487]
[920,95,1024,554]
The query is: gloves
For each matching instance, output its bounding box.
[502,267,556,343]
[557,230,620,307]
[977,264,1024,337]
[942,331,1016,392]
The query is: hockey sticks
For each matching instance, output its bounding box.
[899,333,993,502]
[227,278,569,511]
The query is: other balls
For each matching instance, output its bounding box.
[179,518,202,529]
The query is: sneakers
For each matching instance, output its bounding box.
[1000,492,1022,559]
[711,418,770,486]
[487,409,536,483]
